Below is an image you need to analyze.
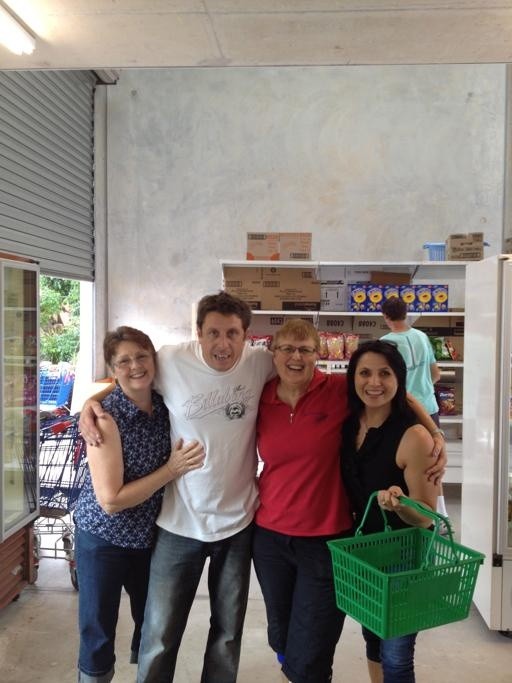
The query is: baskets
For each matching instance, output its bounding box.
[326,491,485,639]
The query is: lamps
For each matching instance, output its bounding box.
[0,5,36,56]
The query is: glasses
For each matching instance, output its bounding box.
[275,344,317,355]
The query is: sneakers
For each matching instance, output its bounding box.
[437,519,455,535]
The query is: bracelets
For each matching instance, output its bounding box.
[430,429,445,437]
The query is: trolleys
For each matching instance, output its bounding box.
[42,413,83,591]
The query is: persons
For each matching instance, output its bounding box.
[72,325,206,682]
[381,296,454,534]
[78,290,278,683]
[338,339,439,683]
[251,318,445,683]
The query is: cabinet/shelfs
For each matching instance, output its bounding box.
[219,260,466,484]
